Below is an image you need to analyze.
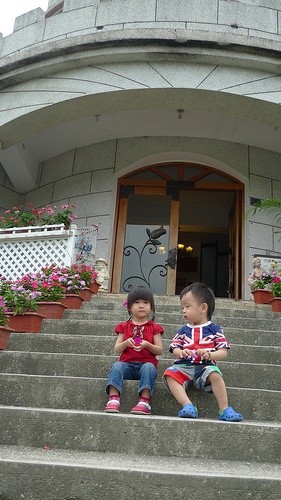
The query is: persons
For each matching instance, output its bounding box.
[94,258,110,288]
[162,282,243,421]
[248,257,267,288]
[105,287,164,414]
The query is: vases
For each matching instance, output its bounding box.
[0,326,15,350]
[82,287,94,302]
[35,302,68,319]
[5,312,46,332]
[251,289,275,304]
[269,297,281,312]
[90,282,101,293]
[57,294,86,309]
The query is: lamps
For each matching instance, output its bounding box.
[177,244,193,264]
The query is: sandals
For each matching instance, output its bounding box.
[177,403,198,419]
[219,407,242,421]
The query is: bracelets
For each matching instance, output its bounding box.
[208,353,210,358]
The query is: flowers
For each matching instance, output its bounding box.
[249,269,281,297]
[135,337,142,346]
[0,253,98,326]
[0,201,77,229]
[123,300,128,309]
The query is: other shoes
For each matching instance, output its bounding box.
[104,399,120,413]
[130,402,152,415]
